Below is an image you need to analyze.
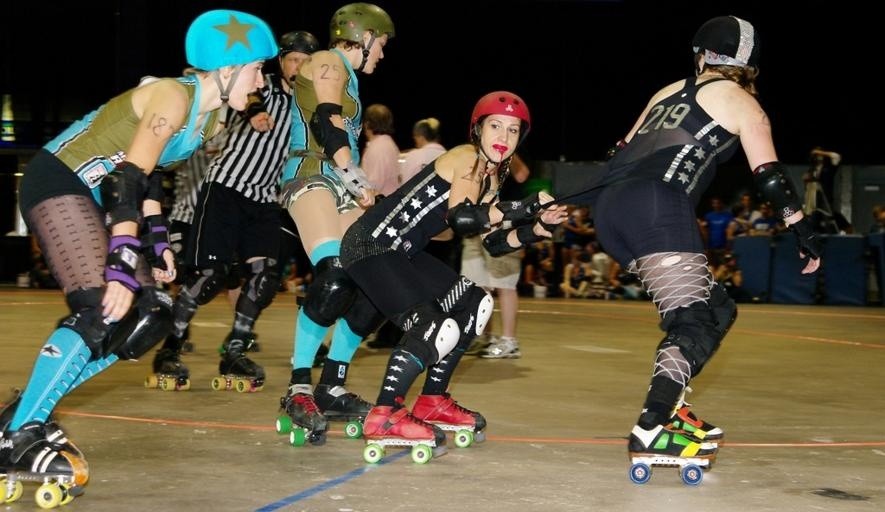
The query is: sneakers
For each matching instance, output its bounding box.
[482,335,520,359]
[290,342,329,367]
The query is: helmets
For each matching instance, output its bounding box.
[470,92,531,150]
[692,15,760,70]
[186,4,396,71]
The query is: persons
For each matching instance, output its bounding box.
[698,150,884,305]
[587,14,823,460]
[144,30,320,392]
[338,91,573,440]
[462,154,529,361]
[520,208,652,301]
[360,107,446,348]
[30,235,61,289]
[0,8,281,488]
[277,4,395,436]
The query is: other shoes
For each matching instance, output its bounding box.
[464,340,485,355]
[180,342,192,351]
[245,331,259,351]
[367,320,406,349]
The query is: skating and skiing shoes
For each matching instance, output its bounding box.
[0,390,88,509]
[627,403,724,485]
[411,386,487,448]
[145,347,191,390]
[275,383,327,447]
[362,396,448,464]
[212,351,266,392]
[312,383,377,438]
